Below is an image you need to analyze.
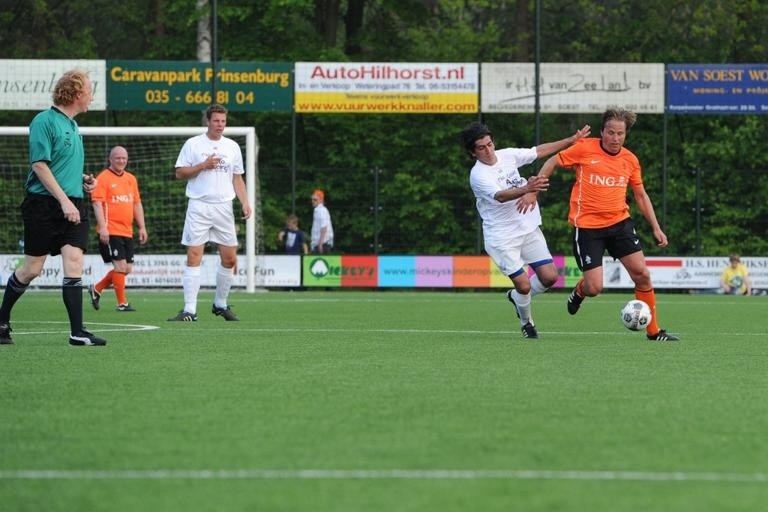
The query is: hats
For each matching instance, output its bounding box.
[312,189,325,202]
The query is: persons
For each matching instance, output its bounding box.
[89,145,147,312]
[170,105,253,322]
[716,254,751,297]
[0,71,107,346]
[311,189,335,255]
[277,216,310,255]
[514,110,679,341]
[461,124,589,338]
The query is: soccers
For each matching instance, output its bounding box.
[619,300,651,331]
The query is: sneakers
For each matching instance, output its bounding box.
[166,310,200,322]
[114,302,137,311]
[567,278,586,315]
[88,283,100,311]
[0,316,14,345]
[507,289,520,319]
[211,302,240,321]
[69,330,106,346]
[646,329,681,341]
[521,323,538,338]
